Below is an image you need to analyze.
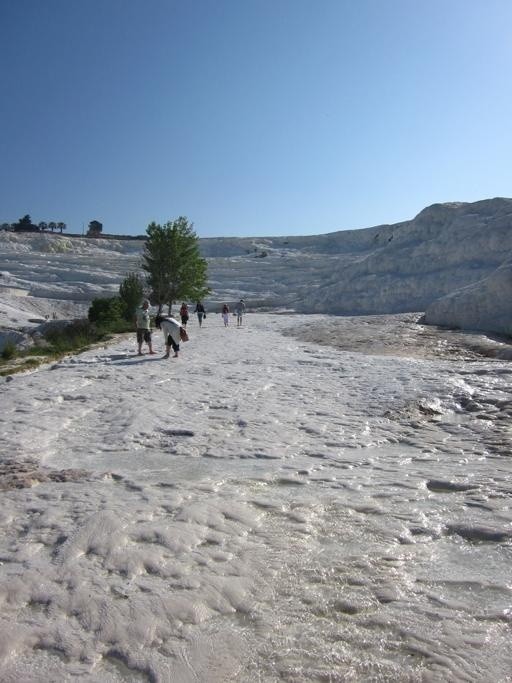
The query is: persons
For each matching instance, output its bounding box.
[222,300,246,327]
[137,300,189,359]
[193,300,206,326]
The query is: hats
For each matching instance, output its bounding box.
[142,300,152,307]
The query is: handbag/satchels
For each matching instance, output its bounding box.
[203,314,206,319]
[180,326,189,342]
